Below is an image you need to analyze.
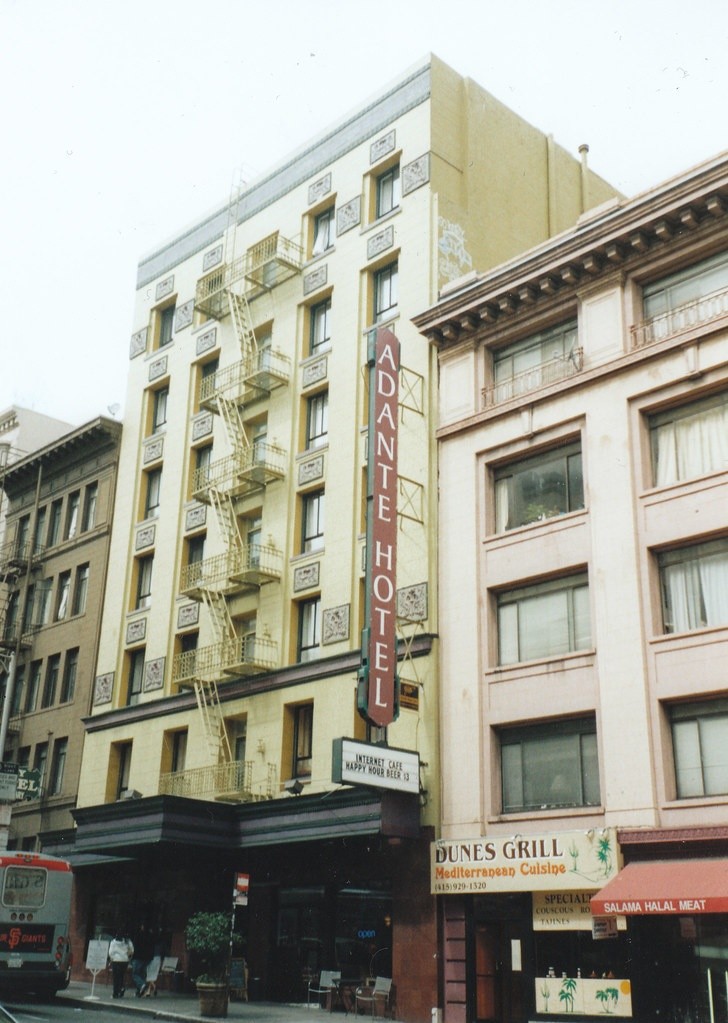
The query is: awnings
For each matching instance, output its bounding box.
[590,857,728,917]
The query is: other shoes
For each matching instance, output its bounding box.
[139,984,146,994]
[119,988,124,997]
[153,986,157,995]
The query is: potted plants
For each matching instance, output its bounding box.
[184,911,245,1016]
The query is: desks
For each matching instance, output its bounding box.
[332,978,369,1017]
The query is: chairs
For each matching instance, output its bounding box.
[305,977,391,1021]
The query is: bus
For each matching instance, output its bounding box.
[0,850,74,1005]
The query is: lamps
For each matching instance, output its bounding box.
[124,790,144,800]
[285,779,304,797]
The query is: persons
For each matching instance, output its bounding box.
[143,923,162,997]
[109,928,135,999]
